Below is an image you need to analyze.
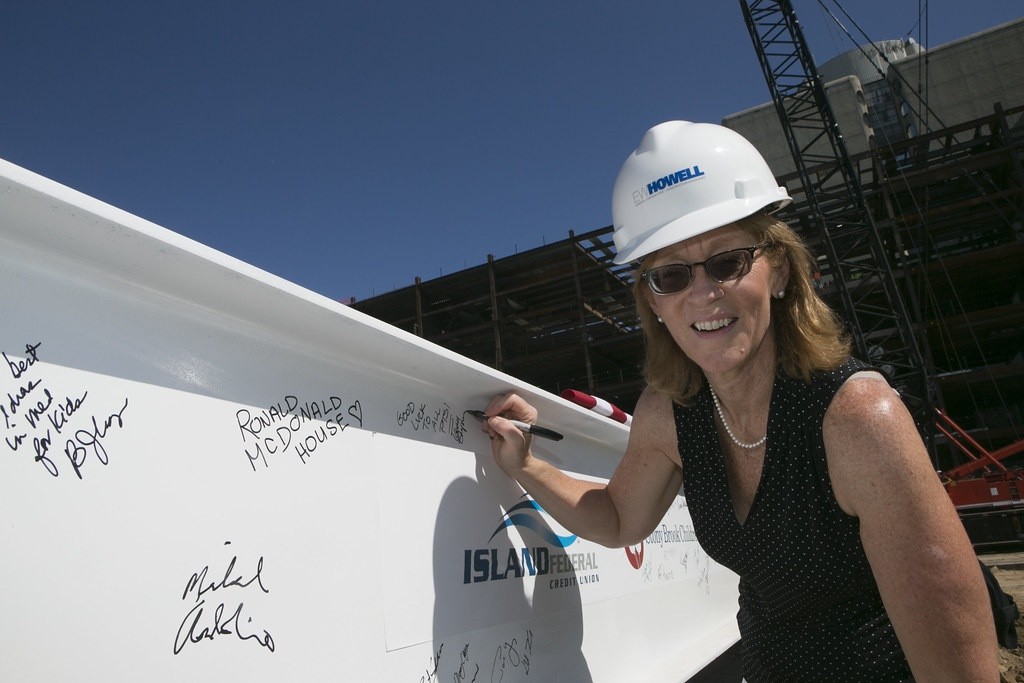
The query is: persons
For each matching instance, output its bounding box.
[481,120,997,683]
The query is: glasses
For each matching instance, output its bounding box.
[640,242,776,295]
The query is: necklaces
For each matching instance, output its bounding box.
[708,380,768,448]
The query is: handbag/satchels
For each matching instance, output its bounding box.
[979,559,1020,648]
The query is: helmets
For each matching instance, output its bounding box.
[612,120,793,265]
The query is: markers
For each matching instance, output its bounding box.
[466,409,564,442]
